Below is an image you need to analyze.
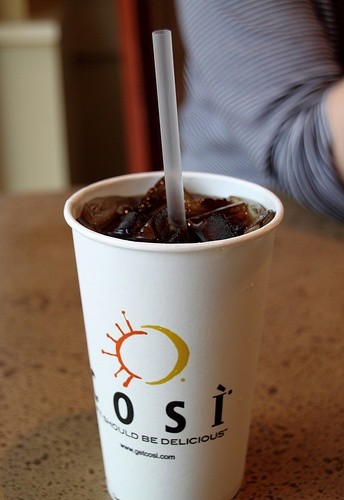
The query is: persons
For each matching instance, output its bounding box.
[173,0,344,226]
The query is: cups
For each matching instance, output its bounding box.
[64,170,284,500]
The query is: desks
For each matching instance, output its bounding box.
[0,188,344,500]
